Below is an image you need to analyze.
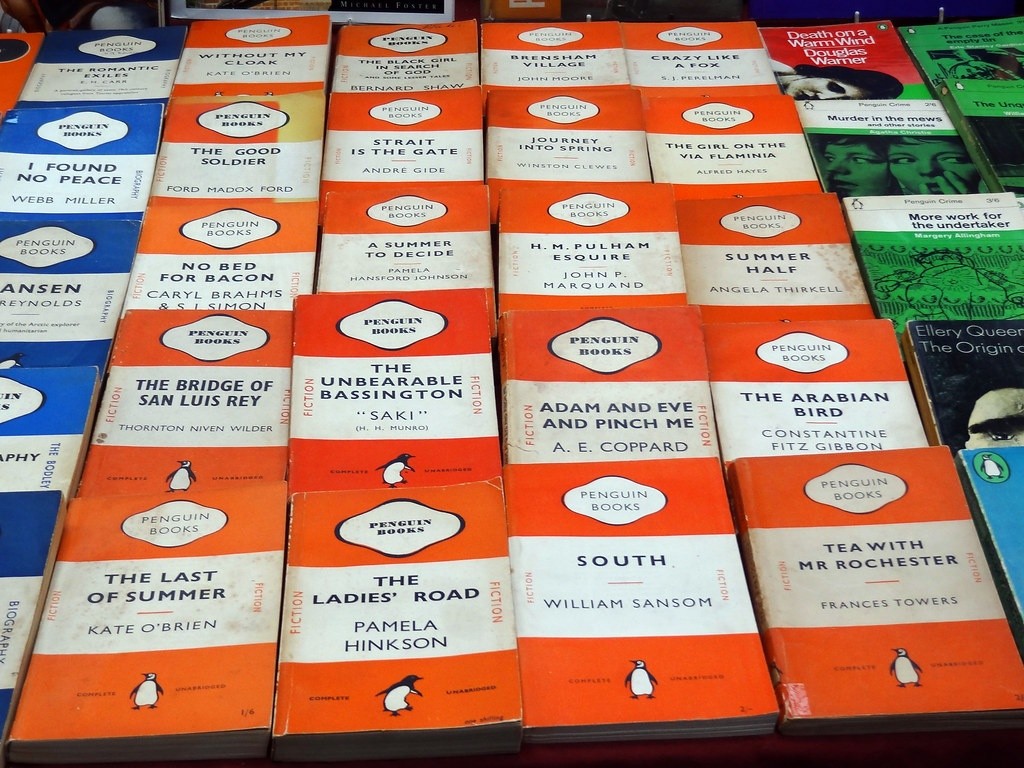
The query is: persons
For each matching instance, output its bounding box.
[820,135,981,196]
[769,56,903,100]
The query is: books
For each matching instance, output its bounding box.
[0,0,1024,765]
